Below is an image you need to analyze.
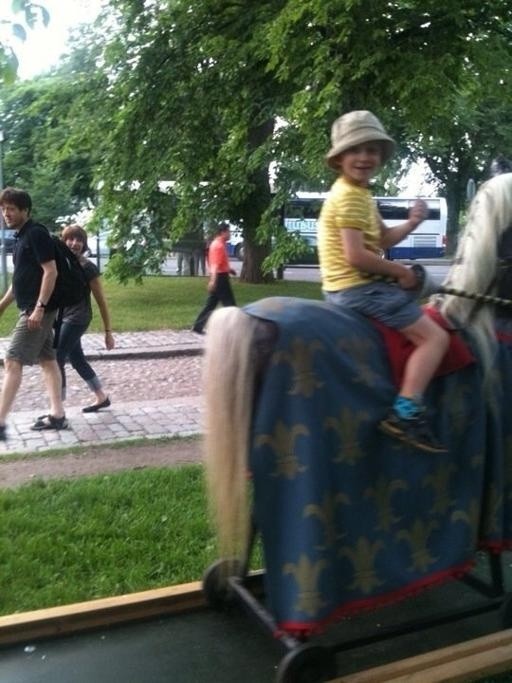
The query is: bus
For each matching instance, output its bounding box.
[218,190,448,261]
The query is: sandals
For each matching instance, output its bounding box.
[31,414,67,430]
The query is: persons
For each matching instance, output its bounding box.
[314,108,461,453]
[1,183,72,443]
[53,222,116,416]
[186,219,242,336]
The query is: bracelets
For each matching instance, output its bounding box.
[104,328,113,332]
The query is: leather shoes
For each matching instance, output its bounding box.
[82,398,110,412]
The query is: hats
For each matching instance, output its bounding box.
[326,110,397,173]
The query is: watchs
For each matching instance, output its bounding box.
[36,299,49,309]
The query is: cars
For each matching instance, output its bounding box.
[83,230,146,258]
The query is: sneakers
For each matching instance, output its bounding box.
[380,406,441,452]
[192,328,205,334]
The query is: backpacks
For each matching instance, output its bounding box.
[49,234,90,309]
